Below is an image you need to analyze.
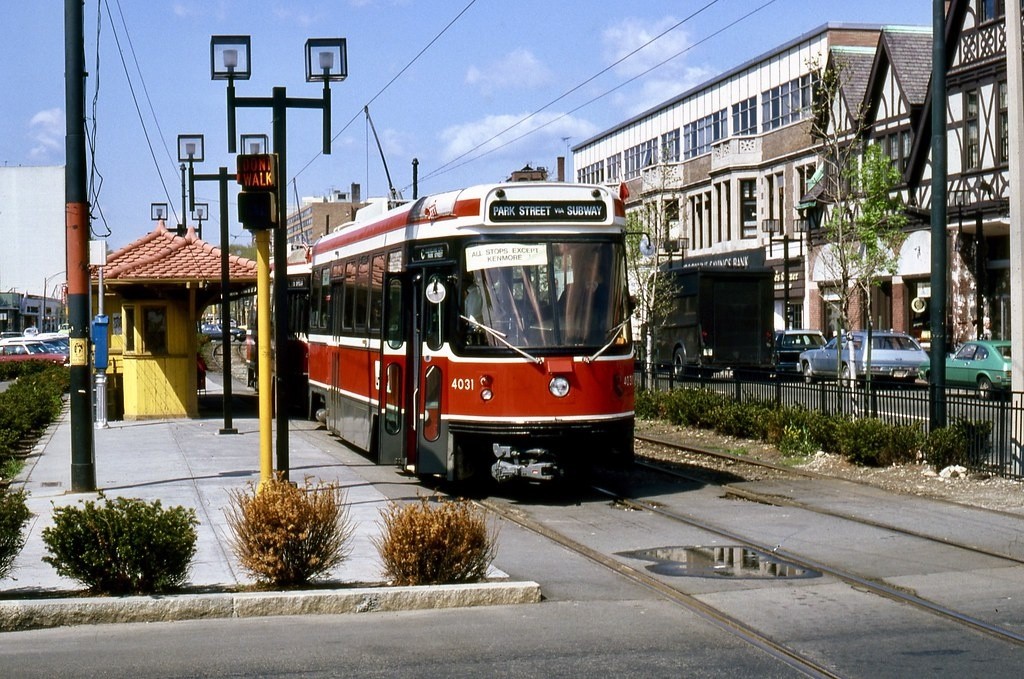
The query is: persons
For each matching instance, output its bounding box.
[464,279,497,328]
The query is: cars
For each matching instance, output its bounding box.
[201,323,246,342]
[0,333,70,365]
[800,331,931,384]
[918,340,1013,399]
[772,329,826,373]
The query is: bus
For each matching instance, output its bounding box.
[623,266,775,380]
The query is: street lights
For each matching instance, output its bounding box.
[151,202,208,239]
[764,218,810,327]
[658,238,690,266]
[211,34,347,496]
[178,134,268,434]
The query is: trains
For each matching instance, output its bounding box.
[247,158,656,487]
